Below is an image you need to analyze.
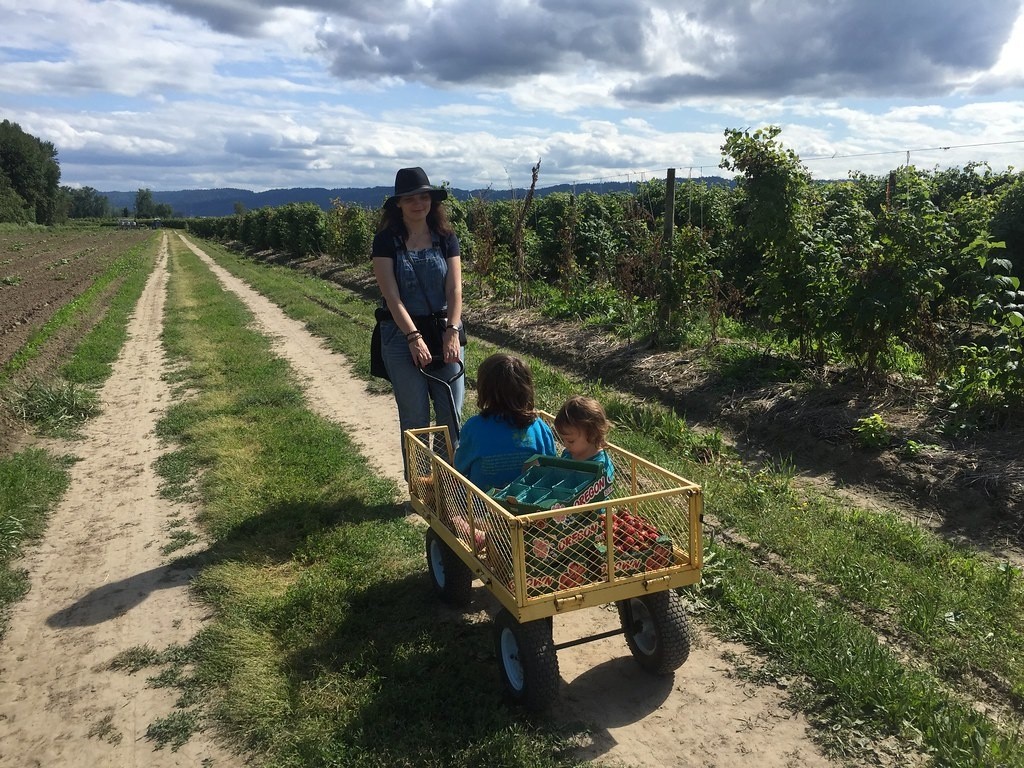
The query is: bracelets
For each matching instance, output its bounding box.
[405,330,422,344]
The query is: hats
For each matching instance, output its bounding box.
[383,167,447,211]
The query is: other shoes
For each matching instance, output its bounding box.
[452,515,486,554]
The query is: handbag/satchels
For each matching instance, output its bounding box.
[431,318,467,349]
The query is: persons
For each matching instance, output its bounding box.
[454,353,615,521]
[370,166,468,485]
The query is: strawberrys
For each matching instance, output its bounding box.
[559,563,586,588]
[599,505,658,555]
[647,545,672,570]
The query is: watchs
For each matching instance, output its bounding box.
[446,325,460,332]
